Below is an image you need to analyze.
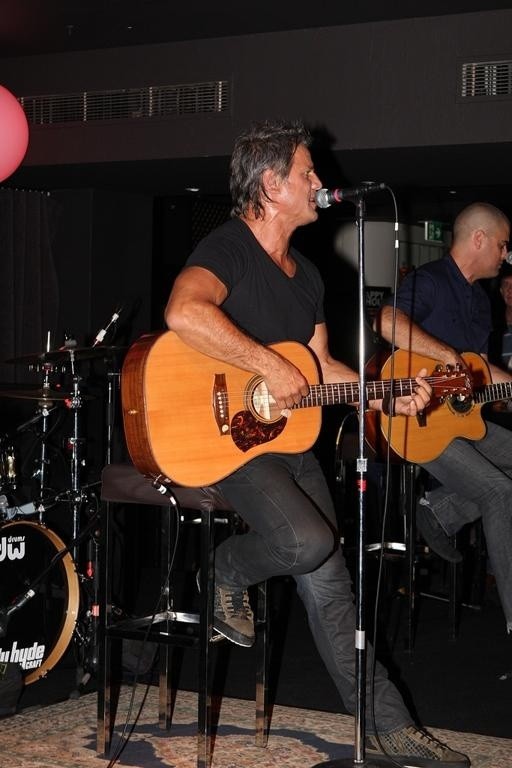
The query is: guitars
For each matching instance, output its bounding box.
[361,342,511,465]
[120,330,471,489]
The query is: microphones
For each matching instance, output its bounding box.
[506,251,512,265]
[315,181,386,209]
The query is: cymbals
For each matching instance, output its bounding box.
[7,344,112,365]
[17,407,56,430]
[3,387,92,402]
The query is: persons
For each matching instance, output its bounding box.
[498,266,512,332]
[162,116,472,768]
[369,201,512,636]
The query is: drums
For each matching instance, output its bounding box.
[2,520,81,687]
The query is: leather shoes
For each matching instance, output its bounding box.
[413,495,464,564]
[196,565,256,649]
[364,726,471,768]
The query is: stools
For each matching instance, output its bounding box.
[92,464,275,768]
[327,428,461,665]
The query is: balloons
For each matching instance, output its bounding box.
[0,85,30,184]
